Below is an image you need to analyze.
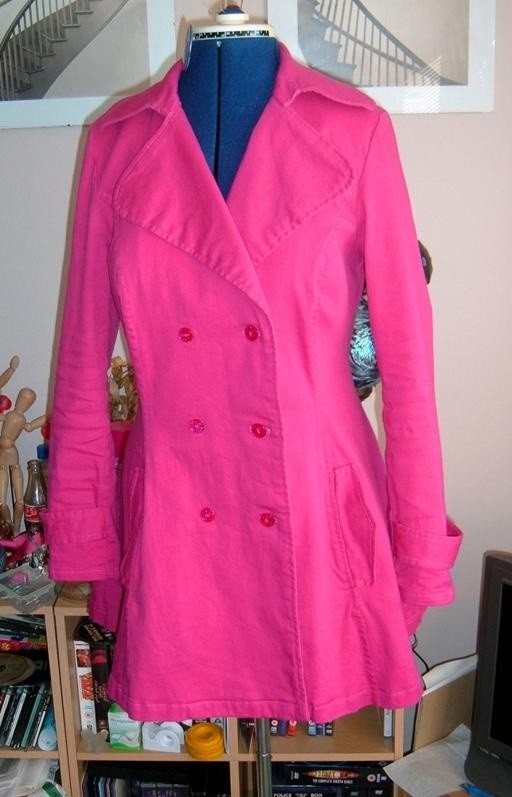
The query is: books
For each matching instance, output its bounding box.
[1,615,56,752]
[239,761,397,796]
[79,762,190,796]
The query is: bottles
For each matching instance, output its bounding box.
[23,444,50,542]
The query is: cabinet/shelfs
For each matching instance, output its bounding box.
[0,580,409,795]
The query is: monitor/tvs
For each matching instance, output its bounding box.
[464,550,512,797]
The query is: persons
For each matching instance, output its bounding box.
[39,2,466,726]
